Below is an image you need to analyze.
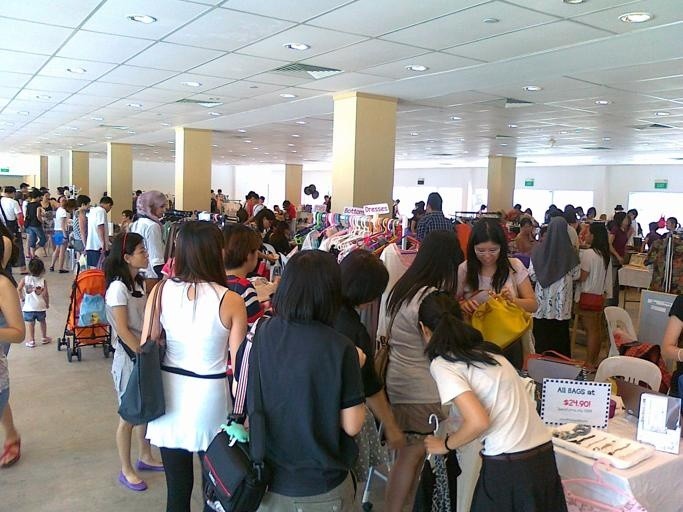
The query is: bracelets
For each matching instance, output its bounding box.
[445,433,453,452]
[678,349,681,361]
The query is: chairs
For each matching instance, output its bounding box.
[66,225,78,269]
[603,306,640,357]
[592,357,663,392]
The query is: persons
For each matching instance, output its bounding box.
[220,224,275,405]
[102,232,166,490]
[333,249,407,511]
[661,295,682,399]
[376,229,465,512]
[418,290,569,512]
[0,235,25,468]
[141,220,247,511]
[0,182,683,370]
[234,249,365,511]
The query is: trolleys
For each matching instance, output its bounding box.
[360,418,395,512]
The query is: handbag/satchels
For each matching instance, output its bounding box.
[457,288,534,351]
[198,315,270,512]
[372,303,404,384]
[0,205,18,233]
[522,348,587,386]
[115,281,167,426]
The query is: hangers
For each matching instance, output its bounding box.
[192,210,227,228]
[294,211,421,257]
[419,413,450,481]
[559,461,632,512]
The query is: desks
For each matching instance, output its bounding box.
[618,267,651,311]
[539,401,683,512]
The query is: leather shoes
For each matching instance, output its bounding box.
[49,266,54,271]
[59,269,70,273]
[118,469,147,491]
[134,457,164,472]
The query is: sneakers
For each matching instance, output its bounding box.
[41,336,52,344]
[19,252,51,275]
[25,341,35,347]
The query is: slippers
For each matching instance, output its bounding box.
[0,435,21,467]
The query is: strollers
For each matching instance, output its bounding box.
[55,260,116,363]
